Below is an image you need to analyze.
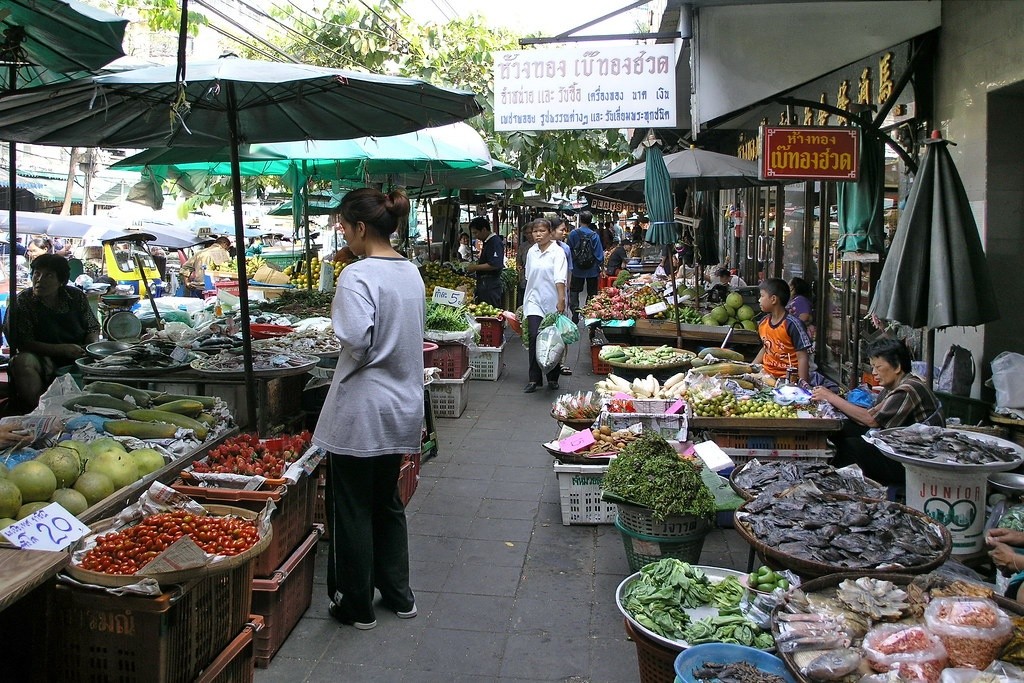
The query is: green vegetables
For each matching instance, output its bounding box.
[425,301,481,344]
[617,269,638,286]
[601,429,716,521]
[619,557,774,649]
[263,288,335,318]
[501,267,519,294]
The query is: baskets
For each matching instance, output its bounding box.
[614,503,709,537]
[733,493,953,574]
[729,459,887,511]
[614,513,708,574]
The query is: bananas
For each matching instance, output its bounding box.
[209,256,267,274]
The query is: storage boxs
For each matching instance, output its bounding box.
[932,391,992,426]
[56,439,420,683]
[203,254,305,307]
[422,310,506,419]
[554,457,618,526]
[589,338,631,375]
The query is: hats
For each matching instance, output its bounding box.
[621,239,633,246]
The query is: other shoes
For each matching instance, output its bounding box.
[523,379,543,393]
[328,601,377,630]
[547,379,559,390]
[382,586,417,619]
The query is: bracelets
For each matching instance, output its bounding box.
[1013,554,1020,573]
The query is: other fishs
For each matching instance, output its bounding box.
[88,336,342,371]
[693,421,1024,683]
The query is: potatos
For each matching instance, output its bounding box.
[577,426,696,462]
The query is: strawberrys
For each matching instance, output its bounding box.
[191,434,303,478]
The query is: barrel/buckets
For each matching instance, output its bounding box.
[902,465,989,554]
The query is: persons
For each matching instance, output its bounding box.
[750,276,813,383]
[246,237,261,256]
[515,218,574,393]
[0,232,28,255]
[985,528,1024,603]
[506,232,513,248]
[54,238,62,250]
[26,237,53,287]
[180,236,232,298]
[464,217,504,309]
[458,233,479,262]
[588,219,642,276]
[312,187,427,630]
[459,225,463,234]
[811,336,947,485]
[513,233,518,249]
[3,254,101,414]
[654,235,747,292]
[568,210,606,325]
[16,236,22,245]
[0,422,37,449]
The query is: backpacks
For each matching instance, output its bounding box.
[570,229,596,269]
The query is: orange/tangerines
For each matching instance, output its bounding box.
[681,387,797,418]
[642,294,674,318]
[422,261,477,302]
[284,257,348,289]
[469,302,501,317]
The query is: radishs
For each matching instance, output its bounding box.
[598,372,687,400]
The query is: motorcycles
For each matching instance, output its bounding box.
[104,241,171,301]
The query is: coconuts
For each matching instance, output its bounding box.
[677,284,705,300]
[0,438,166,542]
[702,293,755,331]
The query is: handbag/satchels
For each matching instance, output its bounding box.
[535,311,580,375]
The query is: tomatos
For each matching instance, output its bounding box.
[77,510,259,574]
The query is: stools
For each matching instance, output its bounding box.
[886,482,906,502]
[420,384,440,468]
[598,271,618,291]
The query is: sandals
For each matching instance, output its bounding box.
[559,364,573,375]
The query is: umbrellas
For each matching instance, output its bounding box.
[578,144,800,304]
[645,132,682,350]
[837,129,884,387]
[865,129,996,393]
[0,0,573,417]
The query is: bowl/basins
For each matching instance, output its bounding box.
[249,323,293,339]
[675,644,795,683]
[988,473,1024,494]
[423,342,438,364]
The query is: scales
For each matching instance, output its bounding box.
[101,295,142,341]
[985,473,1024,522]
